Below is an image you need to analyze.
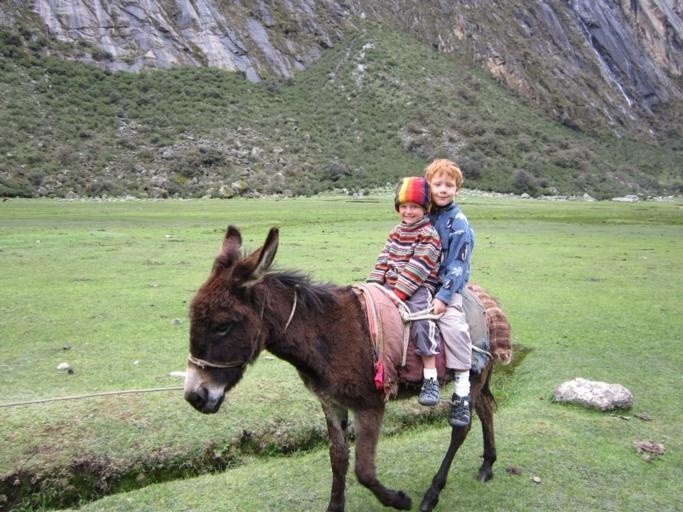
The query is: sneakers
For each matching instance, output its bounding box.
[417,378,440,406]
[448,392,470,429]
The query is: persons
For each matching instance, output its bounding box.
[366,176,442,406]
[424,158,474,427]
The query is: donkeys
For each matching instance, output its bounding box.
[183,225,497,512]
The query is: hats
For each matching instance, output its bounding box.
[394,176,431,212]
